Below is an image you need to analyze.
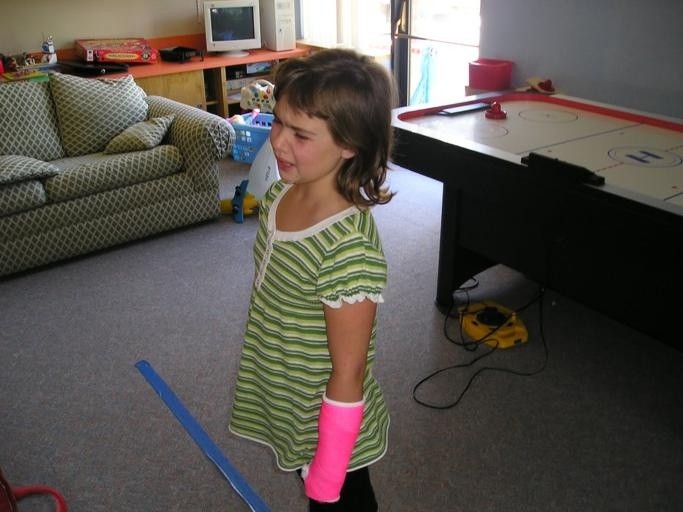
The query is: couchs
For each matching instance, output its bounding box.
[0,80,238,286]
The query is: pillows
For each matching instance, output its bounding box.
[0,69,181,188]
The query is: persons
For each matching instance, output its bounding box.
[228,47,399,512]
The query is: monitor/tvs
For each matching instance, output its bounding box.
[203,0,261,57]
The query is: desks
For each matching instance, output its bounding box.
[390,84,682,351]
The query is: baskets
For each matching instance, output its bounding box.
[231,112,275,164]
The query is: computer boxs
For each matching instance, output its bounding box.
[259,0,296,52]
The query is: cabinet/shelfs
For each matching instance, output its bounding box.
[63,44,311,120]
